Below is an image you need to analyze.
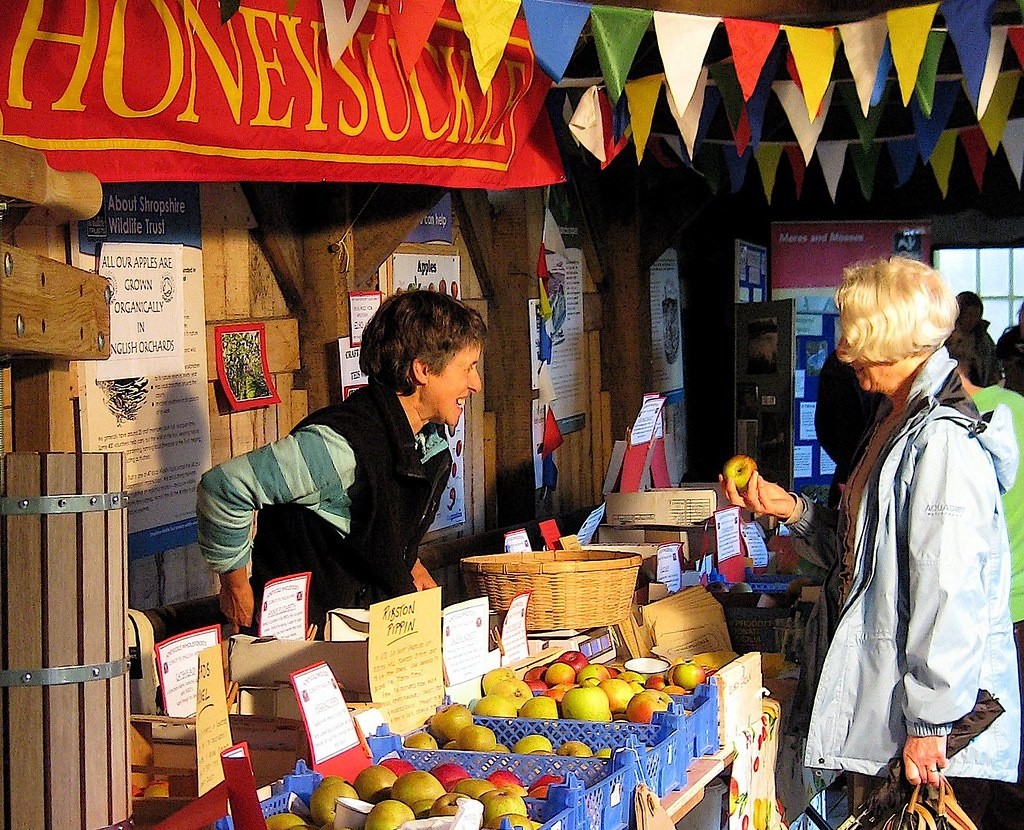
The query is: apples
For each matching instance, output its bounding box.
[724,454,757,493]
[264,651,719,830]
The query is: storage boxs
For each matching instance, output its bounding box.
[717,570,815,651]
[597,480,749,566]
[148,608,762,830]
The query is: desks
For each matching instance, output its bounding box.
[657,749,735,822]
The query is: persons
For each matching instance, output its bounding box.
[195,290,489,640]
[718,254,1024,830]
[814,292,1024,830]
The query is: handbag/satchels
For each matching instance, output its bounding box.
[871,773,977,830]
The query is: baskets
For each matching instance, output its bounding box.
[210,676,719,830]
[460,549,643,630]
